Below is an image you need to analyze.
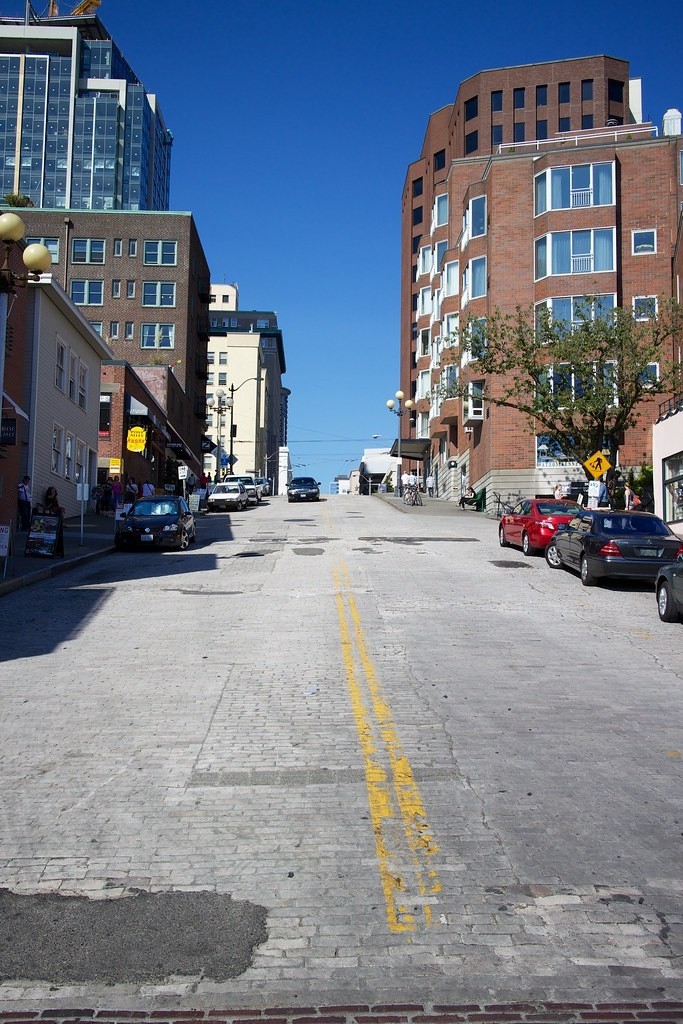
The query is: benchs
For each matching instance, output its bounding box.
[465,487,485,511]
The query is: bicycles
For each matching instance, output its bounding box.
[403,482,423,507]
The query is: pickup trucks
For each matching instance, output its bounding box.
[224,473,263,506]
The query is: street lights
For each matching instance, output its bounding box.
[1,212,53,407]
[228,377,265,476]
[386,390,414,498]
[263,450,290,481]
[206,388,234,484]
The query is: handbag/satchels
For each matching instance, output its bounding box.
[632,495,642,506]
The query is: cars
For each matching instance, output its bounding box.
[117,494,198,552]
[544,508,683,586]
[498,497,585,556]
[655,554,683,624]
[285,477,322,502]
[255,477,272,496]
[206,481,250,512]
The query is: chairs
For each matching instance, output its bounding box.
[540,508,551,515]
[567,509,579,515]
[223,488,229,492]
[636,522,657,533]
[245,479,251,483]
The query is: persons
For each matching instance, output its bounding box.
[18,475,32,532]
[623,482,635,511]
[426,474,434,498]
[456,486,475,511]
[554,484,567,500]
[125,477,155,504]
[99,475,122,519]
[31,520,54,532]
[188,472,211,494]
[598,477,609,508]
[401,470,416,490]
[44,486,69,528]
[243,479,249,485]
